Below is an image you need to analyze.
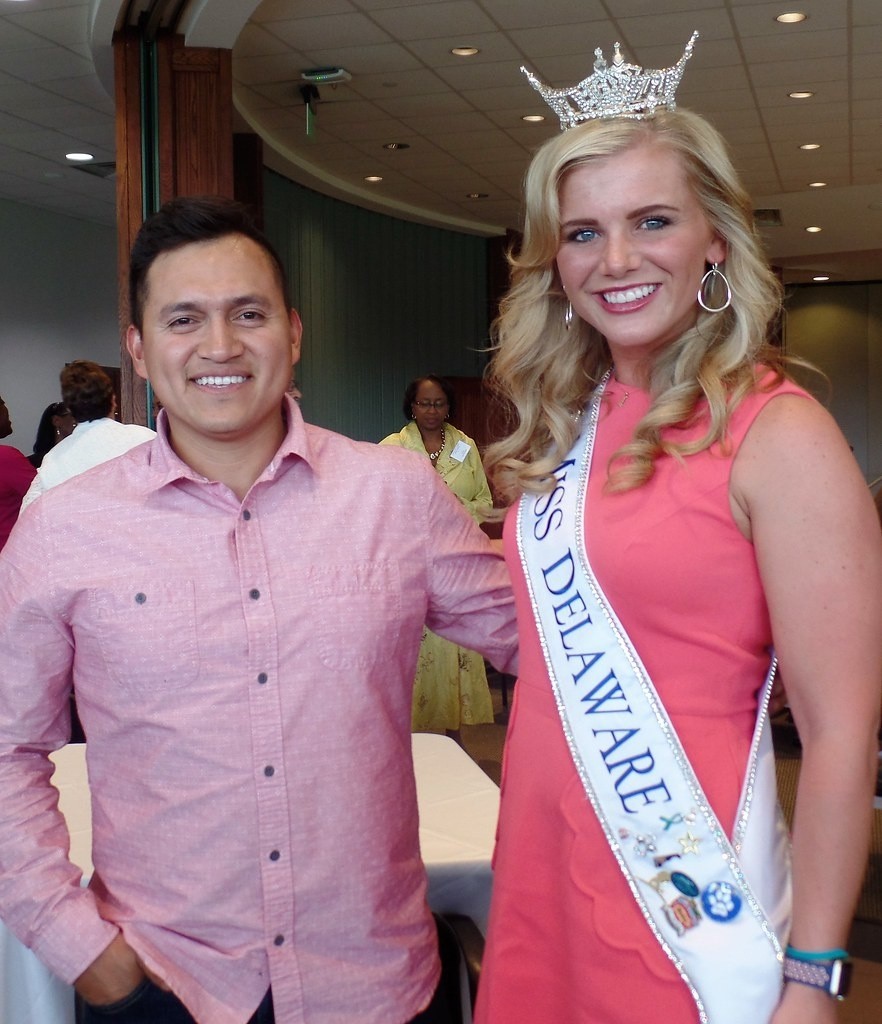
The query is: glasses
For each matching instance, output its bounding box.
[414,400,449,410]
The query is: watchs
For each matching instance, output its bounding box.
[783,961,853,1002]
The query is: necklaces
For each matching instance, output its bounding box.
[429,424,445,459]
[611,372,649,408]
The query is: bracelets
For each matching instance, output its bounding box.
[783,939,850,962]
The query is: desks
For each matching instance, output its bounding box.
[0,732,500,1024]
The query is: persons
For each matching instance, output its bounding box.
[471,31,880,1023]
[373,377,493,739]
[0,362,160,549]
[1,199,521,1024]
